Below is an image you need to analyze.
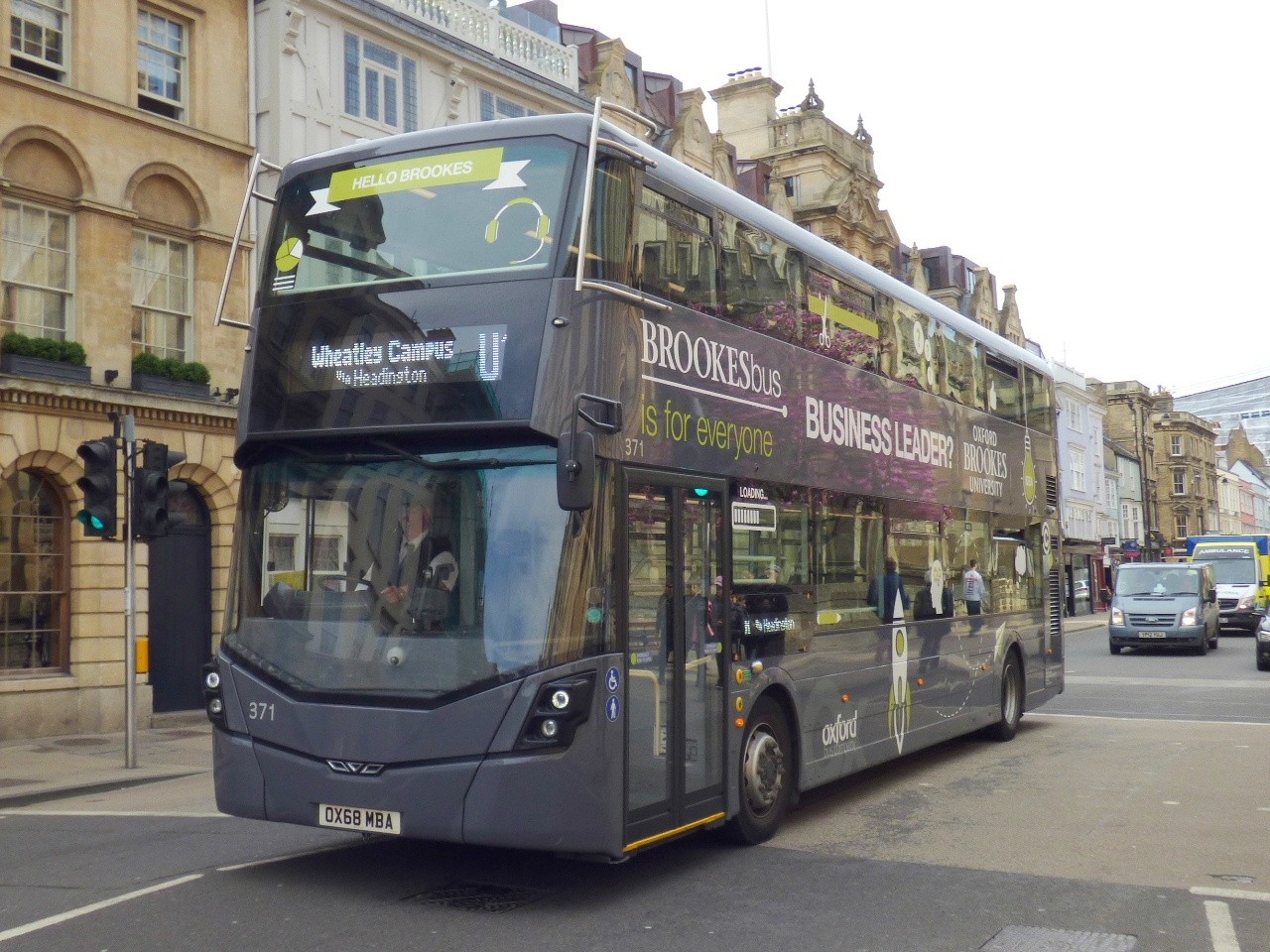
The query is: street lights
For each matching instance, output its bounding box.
[1140,407,1174,562]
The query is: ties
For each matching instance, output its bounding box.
[399,543,414,566]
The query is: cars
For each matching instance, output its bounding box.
[1255,606,1270,671]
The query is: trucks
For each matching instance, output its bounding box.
[1187,533,1270,631]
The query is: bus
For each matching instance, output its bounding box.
[203,111,1065,869]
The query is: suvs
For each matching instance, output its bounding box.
[1107,562,1221,656]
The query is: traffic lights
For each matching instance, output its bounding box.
[76,435,118,537]
[132,442,169,538]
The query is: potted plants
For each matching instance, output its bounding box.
[134,353,210,400]
[0,333,92,382]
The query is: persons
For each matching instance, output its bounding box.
[655,556,987,685]
[355,485,460,604]
